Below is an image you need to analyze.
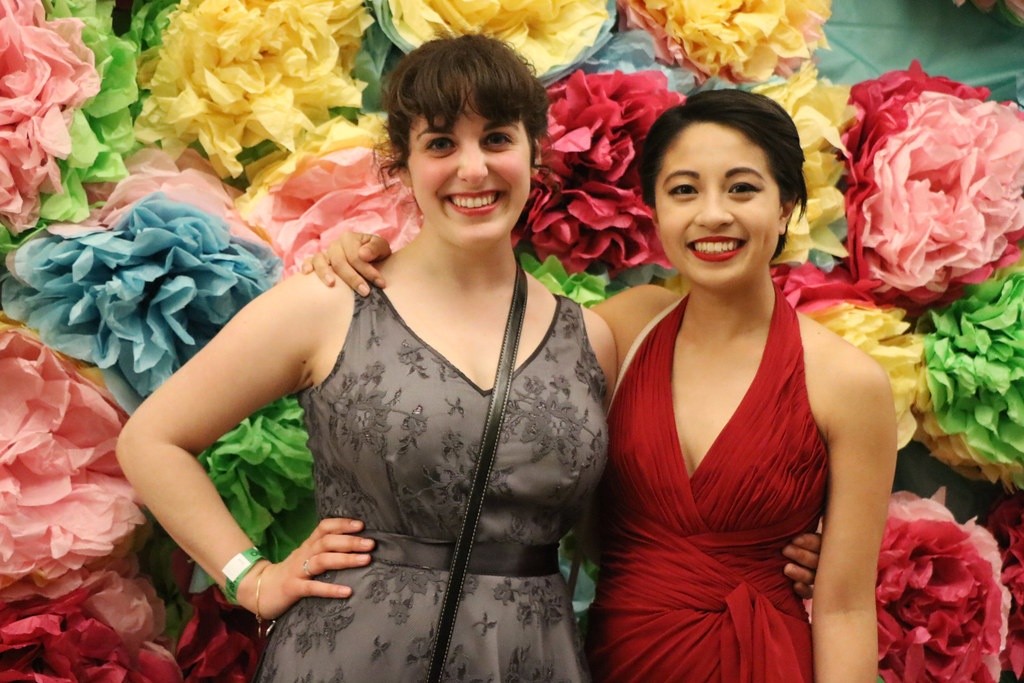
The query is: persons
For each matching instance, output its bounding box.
[116,34,897,683]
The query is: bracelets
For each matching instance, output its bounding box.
[222,546,266,606]
[255,576,262,638]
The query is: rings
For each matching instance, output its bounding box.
[303,559,312,576]
